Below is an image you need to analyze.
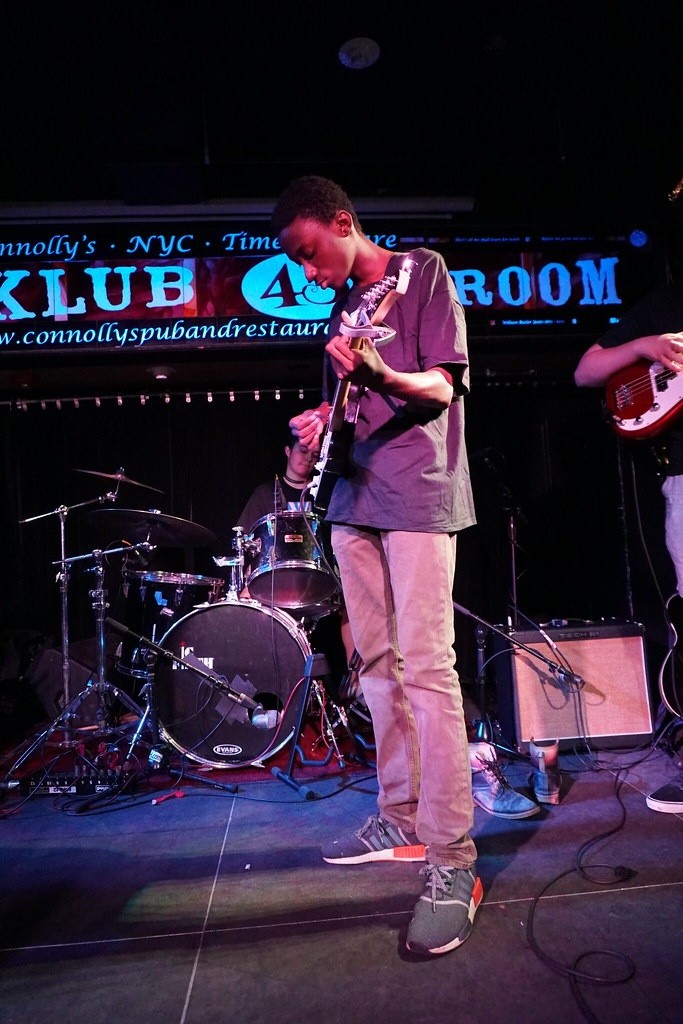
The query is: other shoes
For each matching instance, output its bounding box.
[646,769,683,813]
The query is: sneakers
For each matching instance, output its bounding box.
[320,811,428,864]
[405,863,484,956]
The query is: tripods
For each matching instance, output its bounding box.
[450,599,582,763]
[0,476,263,814]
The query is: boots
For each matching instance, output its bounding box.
[527,738,562,804]
[468,743,541,819]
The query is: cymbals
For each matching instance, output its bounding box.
[79,508,218,548]
[70,467,165,495]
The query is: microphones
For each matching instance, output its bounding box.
[275,475,288,510]
[252,706,281,730]
[559,673,585,685]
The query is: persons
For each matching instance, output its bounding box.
[236,427,374,723]
[273,175,483,957]
[574,283,683,813]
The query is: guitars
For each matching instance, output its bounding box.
[659,593,683,720]
[307,251,417,511]
[606,342,683,439]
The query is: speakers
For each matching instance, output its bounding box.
[493,622,656,753]
[19,648,118,728]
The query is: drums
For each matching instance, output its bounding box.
[114,509,342,770]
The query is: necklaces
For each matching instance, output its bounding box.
[284,475,310,484]
